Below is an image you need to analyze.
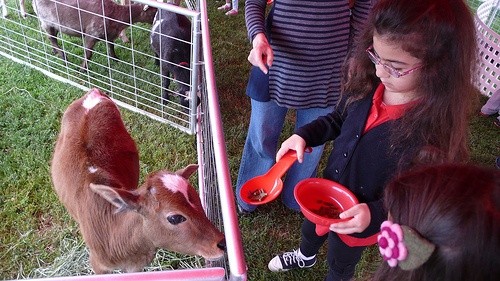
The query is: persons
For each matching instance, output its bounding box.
[217,0,239,16]
[371,163,500,281]
[235,0,372,216]
[268,0,477,281]
[480,88,500,117]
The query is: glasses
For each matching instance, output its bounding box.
[365,44,422,79]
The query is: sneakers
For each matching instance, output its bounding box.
[267,249,319,272]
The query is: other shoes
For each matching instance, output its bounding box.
[237,203,250,216]
[225,9,239,16]
[217,4,231,10]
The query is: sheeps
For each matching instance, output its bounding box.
[0,0,200,112]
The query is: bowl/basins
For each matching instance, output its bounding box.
[294,177,360,228]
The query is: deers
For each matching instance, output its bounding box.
[51,88,227,274]
